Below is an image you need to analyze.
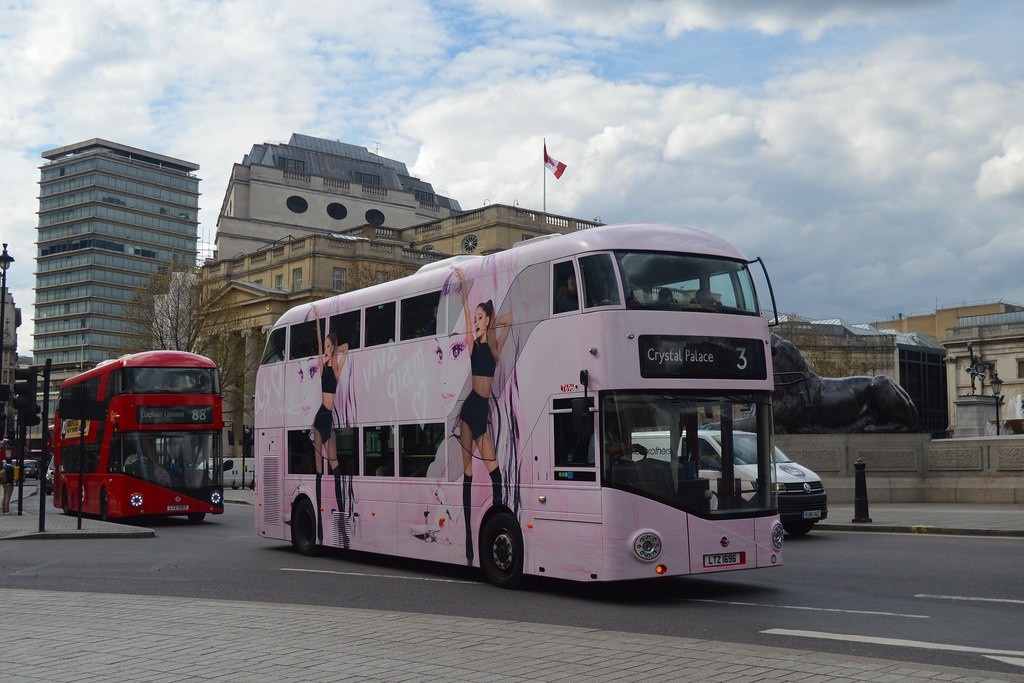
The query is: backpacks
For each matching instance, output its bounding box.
[0,465,11,484]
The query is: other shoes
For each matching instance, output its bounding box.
[5,511,16,514]
[1,512,5,514]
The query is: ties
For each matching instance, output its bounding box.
[608,435,612,473]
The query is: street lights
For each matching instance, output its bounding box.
[990,370,1004,436]
[0,242,14,373]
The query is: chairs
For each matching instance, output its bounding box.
[124,454,151,465]
[553,411,594,464]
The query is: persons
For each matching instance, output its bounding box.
[587,416,647,466]
[658,287,674,302]
[0,459,14,515]
[555,275,577,297]
[454,267,513,565]
[696,287,722,305]
[311,305,350,544]
[124,443,149,466]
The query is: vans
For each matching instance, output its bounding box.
[631,430,828,536]
[197,458,256,491]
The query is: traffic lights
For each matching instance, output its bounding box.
[14,366,40,427]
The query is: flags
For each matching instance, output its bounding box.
[543,145,566,178]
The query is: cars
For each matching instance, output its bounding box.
[45,456,55,495]
[24,460,40,480]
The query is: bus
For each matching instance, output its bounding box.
[255,221,812,593]
[52,349,235,523]
[48,425,53,453]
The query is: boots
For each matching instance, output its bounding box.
[316,471,323,541]
[489,466,502,506]
[332,464,350,548]
[463,473,474,560]
